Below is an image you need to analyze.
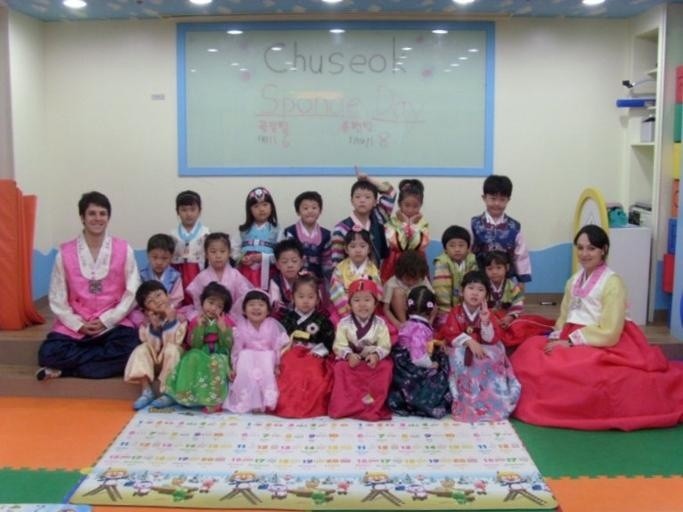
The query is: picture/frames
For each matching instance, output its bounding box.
[172,18,499,179]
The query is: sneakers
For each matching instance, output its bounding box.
[133,392,154,410]
[152,394,174,407]
[37,368,63,381]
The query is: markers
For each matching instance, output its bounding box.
[539,301,556,305]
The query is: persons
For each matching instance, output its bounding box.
[163,280,236,413]
[481,250,529,350]
[124,279,188,410]
[34,190,144,381]
[385,285,453,420]
[221,288,289,414]
[467,174,529,293]
[265,265,336,418]
[136,168,439,345]
[432,225,478,329]
[328,279,394,422]
[444,270,522,423]
[502,224,683,431]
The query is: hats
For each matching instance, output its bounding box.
[349,279,378,303]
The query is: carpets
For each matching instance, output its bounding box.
[64,400,561,512]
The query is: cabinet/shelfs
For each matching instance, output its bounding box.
[623,25,659,217]
[609,222,651,325]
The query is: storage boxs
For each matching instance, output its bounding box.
[640,114,655,142]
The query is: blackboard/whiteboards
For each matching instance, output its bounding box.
[177,19,496,177]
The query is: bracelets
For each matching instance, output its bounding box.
[568,338,574,347]
